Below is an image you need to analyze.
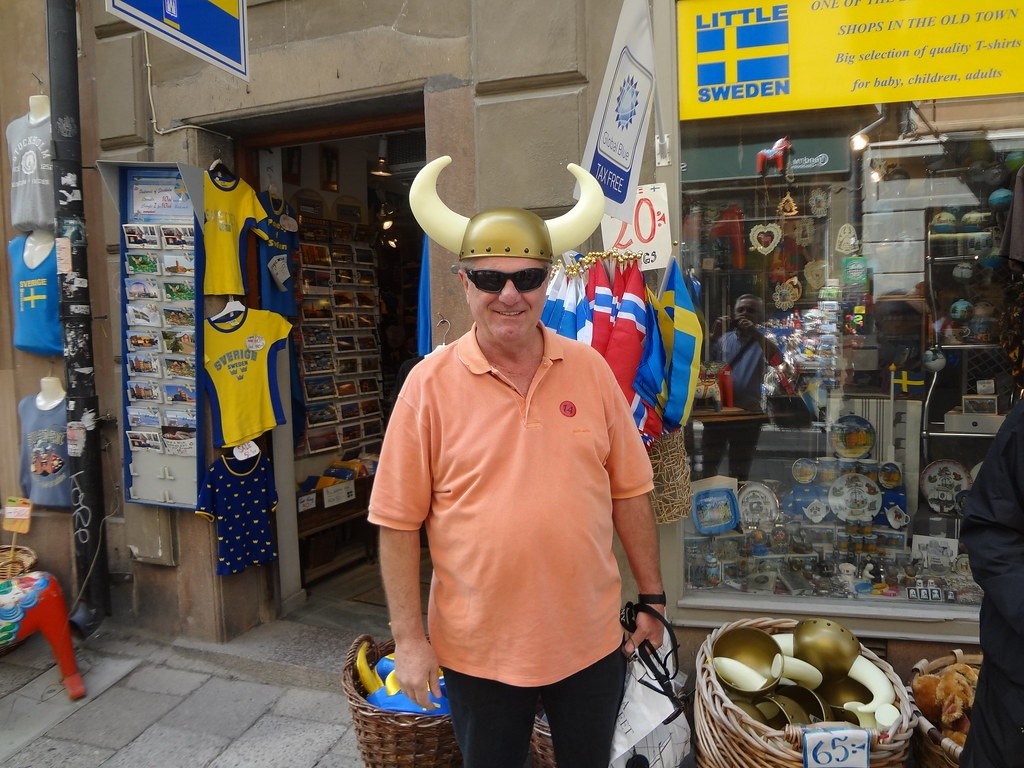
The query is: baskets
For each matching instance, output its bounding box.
[0,544,37,582]
[530,715,557,768]
[643,430,693,525]
[905,648,984,768]
[693,617,918,768]
[341,633,464,768]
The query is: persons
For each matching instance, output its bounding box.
[959,394,1024,768]
[366,157,666,768]
[969,300,999,340]
[704,294,784,491]
[7,95,69,509]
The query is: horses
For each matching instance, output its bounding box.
[756,135,794,174]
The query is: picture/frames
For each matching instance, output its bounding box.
[319,143,339,193]
[281,146,302,187]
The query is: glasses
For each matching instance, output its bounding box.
[465,267,549,294]
[733,305,761,316]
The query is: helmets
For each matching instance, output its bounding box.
[356,642,451,717]
[408,155,606,264]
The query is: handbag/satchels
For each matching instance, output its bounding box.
[604,609,692,767]
[691,364,733,412]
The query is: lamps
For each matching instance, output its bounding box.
[370,135,392,176]
[380,195,397,217]
[849,111,888,154]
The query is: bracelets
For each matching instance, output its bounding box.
[638,592,667,609]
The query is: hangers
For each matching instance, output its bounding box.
[223,446,234,458]
[267,167,282,201]
[208,148,238,182]
[211,294,246,321]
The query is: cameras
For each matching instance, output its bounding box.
[729,319,739,330]
